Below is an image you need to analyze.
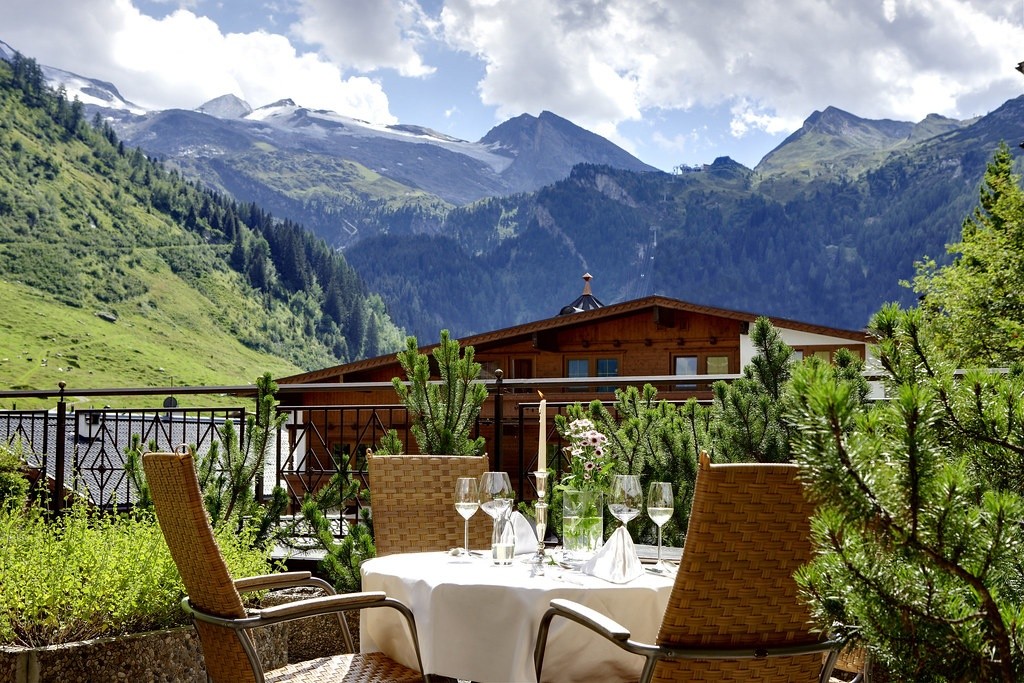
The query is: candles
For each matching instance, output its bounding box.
[537,389,546,478]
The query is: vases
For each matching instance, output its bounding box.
[560,490,604,570]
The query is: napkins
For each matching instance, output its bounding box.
[500,511,540,557]
[580,525,647,585]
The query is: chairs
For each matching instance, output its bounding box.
[365,447,496,559]
[140,451,430,683]
[533,446,853,683]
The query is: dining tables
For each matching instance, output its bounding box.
[358,548,682,683]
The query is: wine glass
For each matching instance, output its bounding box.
[447,477,483,559]
[645,482,674,577]
[478,472,514,567]
[607,474,643,530]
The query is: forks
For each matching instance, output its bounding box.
[531,563,584,586]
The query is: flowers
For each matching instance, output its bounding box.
[562,418,616,551]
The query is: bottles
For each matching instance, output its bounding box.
[490,498,515,565]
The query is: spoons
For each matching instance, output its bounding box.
[661,558,680,572]
